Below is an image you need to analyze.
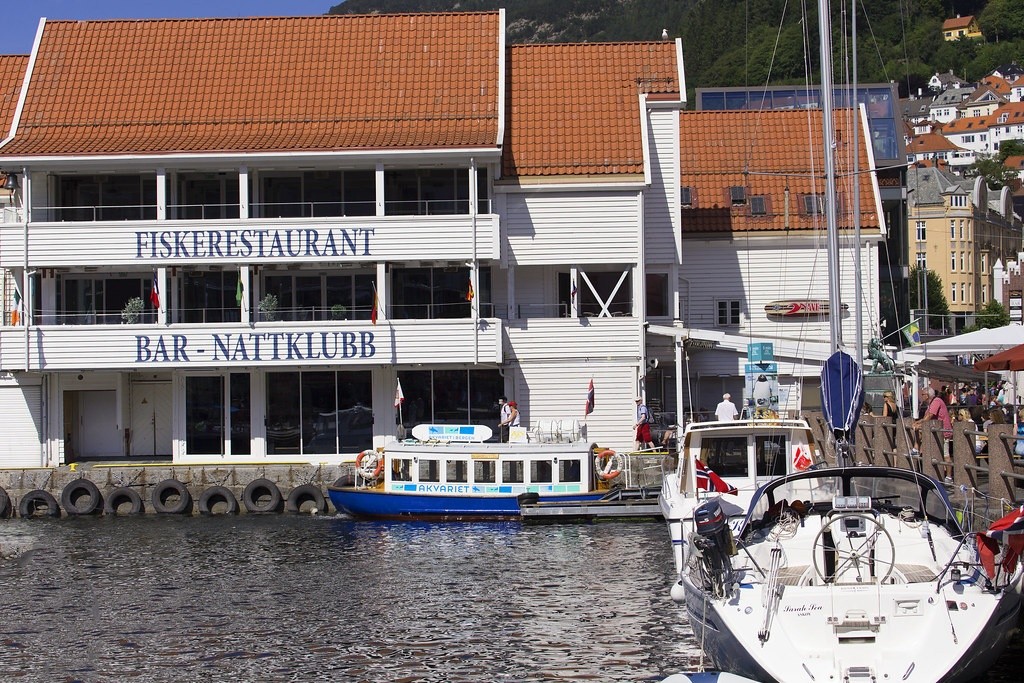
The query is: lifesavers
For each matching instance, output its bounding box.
[19,490,58,518]
[199,485,236,514]
[104,487,141,515]
[151,479,190,514]
[518,493,539,499]
[0,486,8,514]
[61,480,99,515]
[333,475,365,487]
[286,485,325,511]
[596,450,624,478]
[356,449,382,479]
[242,479,281,515]
[517,500,539,504]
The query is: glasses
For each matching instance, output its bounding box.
[922,393,925,396]
[884,395,887,398]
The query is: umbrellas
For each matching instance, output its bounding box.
[896,321,1024,424]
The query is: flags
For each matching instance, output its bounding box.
[585,378,594,415]
[976,504,1024,579]
[372,289,378,324]
[236,273,244,306]
[695,458,738,495]
[901,320,921,346]
[394,379,404,407]
[12,288,21,326]
[466,279,474,301]
[572,281,576,307]
[150,272,160,308]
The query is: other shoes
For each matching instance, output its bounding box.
[907,447,920,457]
[944,477,954,484]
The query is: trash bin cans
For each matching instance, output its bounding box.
[788,409,799,426]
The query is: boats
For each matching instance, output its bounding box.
[325,419,673,522]
[657,418,840,576]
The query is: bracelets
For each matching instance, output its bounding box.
[636,424,638,426]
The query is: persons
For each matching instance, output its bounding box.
[868,380,1024,486]
[633,396,656,452]
[658,407,709,447]
[497,396,521,443]
[713,393,739,466]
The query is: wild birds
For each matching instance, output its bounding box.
[662,28,669,40]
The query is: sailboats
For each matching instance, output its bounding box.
[678,0,1024,683]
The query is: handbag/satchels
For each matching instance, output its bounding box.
[998,390,1005,403]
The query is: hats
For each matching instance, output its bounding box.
[953,382,964,390]
[1001,382,1013,391]
[507,401,517,406]
[635,396,642,402]
